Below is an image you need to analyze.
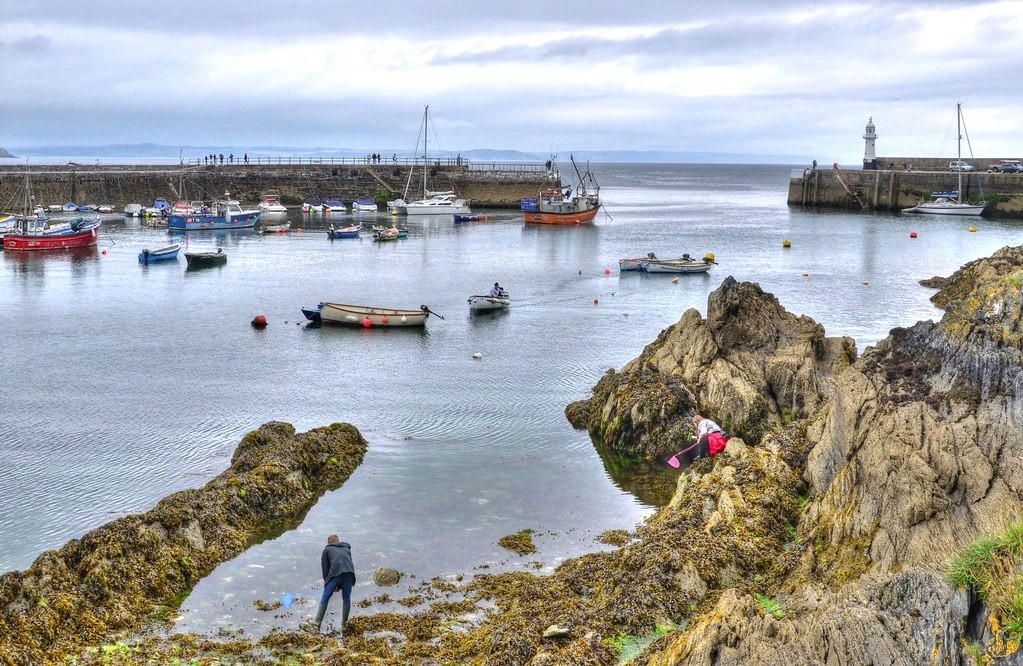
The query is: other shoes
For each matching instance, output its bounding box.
[693,455,706,461]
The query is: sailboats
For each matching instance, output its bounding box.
[0,156,50,246]
[916,101,989,216]
[406,104,472,215]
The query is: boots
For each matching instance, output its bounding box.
[309,603,327,627]
[342,606,351,627]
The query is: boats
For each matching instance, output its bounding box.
[372,228,409,241]
[124,197,207,216]
[453,213,488,223]
[326,221,363,239]
[639,259,712,273]
[522,135,603,224]
[301,198,407,215]
[138,243,181,262]
[3,215,102,252]
[257,194,295,233]
[183,252,227,271]
[167,148,263,228]
[469,295,510,314]
[33,203,116,215]
[619,256,696,270]
[300,300,429,328]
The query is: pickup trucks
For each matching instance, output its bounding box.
[987,163,1023,174]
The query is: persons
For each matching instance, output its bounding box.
[378,153,380,163]
[367,152,371,164]
[491,282,509,297]
[690,415,729,460]
[567,188,571,199]
[492,161,495,169]
[393,153,397,164]
[309,535,356,628]
[206,153,234,164]
[372,153,376,164]
[545,160,548,170]
[548,159,552,170]
[95,159,100,173]
[457,154,461,166]
[244,153,249,164]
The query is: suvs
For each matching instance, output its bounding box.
[949,160,975,172]
